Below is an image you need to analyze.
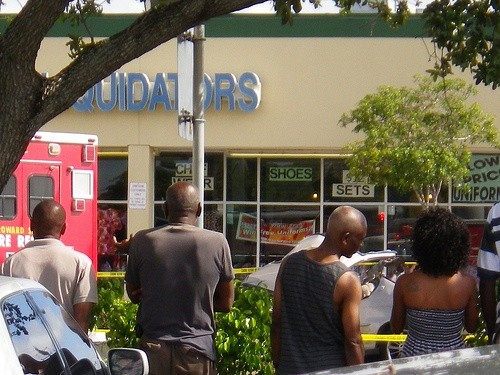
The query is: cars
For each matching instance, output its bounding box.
[0,276,150,375]
[241,251,409,360]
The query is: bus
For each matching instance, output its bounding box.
[0,132,99,283]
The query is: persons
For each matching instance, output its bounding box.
[390,208,479,359]
[125,182,234,375]
[270,206,367,375]
[477,201,500,345]
[0,200,98,336]
